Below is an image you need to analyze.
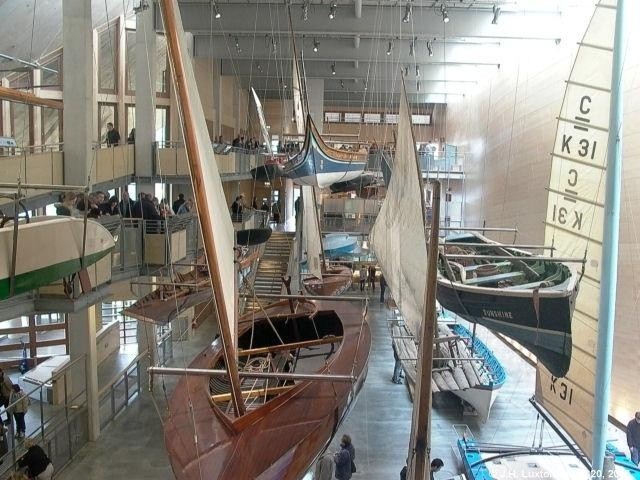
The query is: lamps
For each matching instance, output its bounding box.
[403,3,412,23]
[328,3,338,19]
[440,8,450,23]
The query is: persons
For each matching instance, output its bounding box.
[379,273,388,304]
[214,135,299,155]
[9,383,29,438]
[359,264,367,292]
[55,187,280,233]
[294,195,301,215]
[0,415,9,466]
[626,410,640,466]
[126,127,134,144]
[368,265,376,291]
[332,434,356,479]
[392,344,404,384]
[100,123,120,147]
[430,459,444,480]
[17,437,55,480]
[0,369,15,427]
[400,458,409,480]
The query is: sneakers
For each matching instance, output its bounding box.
[393,375,404,384]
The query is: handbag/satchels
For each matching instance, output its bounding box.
[351,461,356,473]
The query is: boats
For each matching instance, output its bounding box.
[0,170,115,301]
